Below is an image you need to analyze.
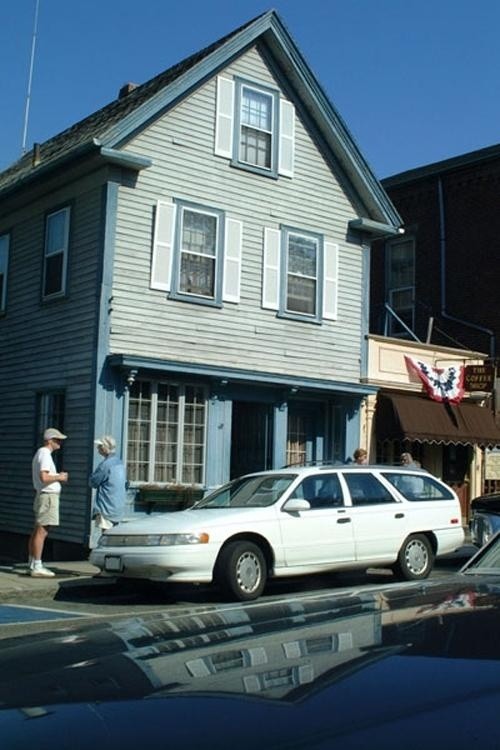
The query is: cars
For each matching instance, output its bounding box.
[88,465,465,601]
[458,492,500,577]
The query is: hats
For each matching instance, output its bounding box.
[94,436,116,450]
[44,428,68,441]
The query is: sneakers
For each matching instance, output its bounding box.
[26,568,56,577]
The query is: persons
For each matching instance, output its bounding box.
[25,428,68,577]
[87,435,127,579]
[399,452,424,498]
[320,447,369,499]
